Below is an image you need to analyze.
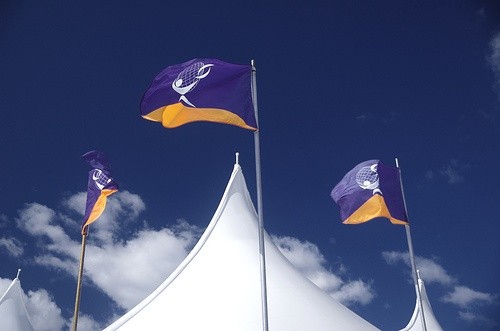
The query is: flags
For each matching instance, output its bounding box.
[329,160,409,225]
[81,151,119,235]
[140,58,259,132]
